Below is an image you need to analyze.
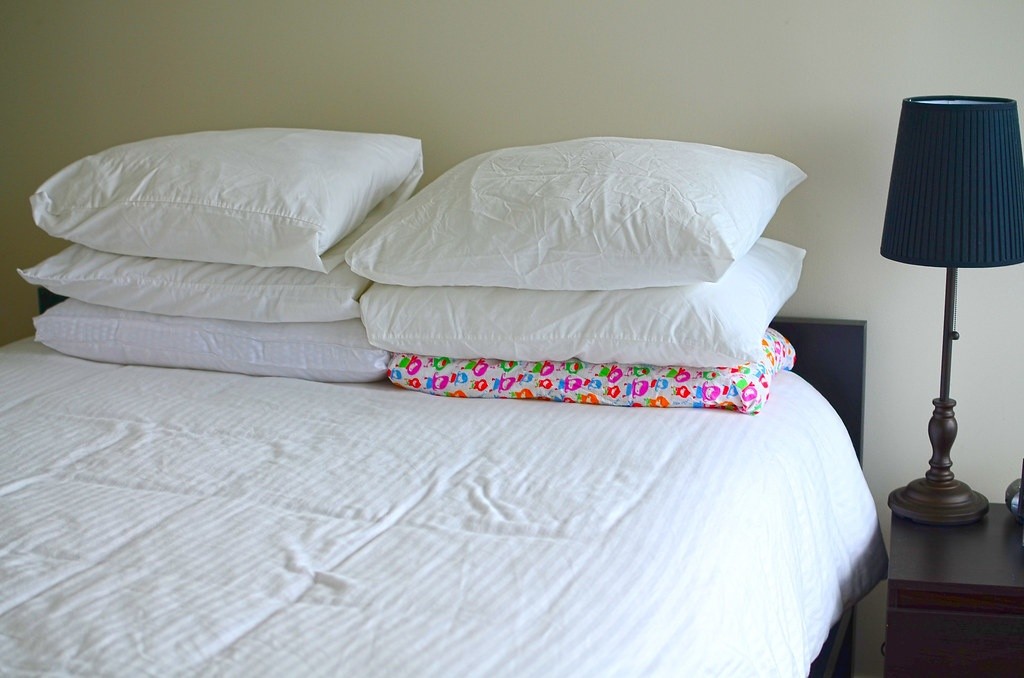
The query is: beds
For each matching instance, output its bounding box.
[0,318,882,678]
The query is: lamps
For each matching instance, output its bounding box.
[879,95,1024,528]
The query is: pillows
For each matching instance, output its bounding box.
[342,134,808,294]
[28,298,391,389]
[382,328,798,420]
[356,237,808,368]
[15,244,364,328]
[28,126,426,276]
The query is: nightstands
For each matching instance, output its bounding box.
[881,503,1024,678]
